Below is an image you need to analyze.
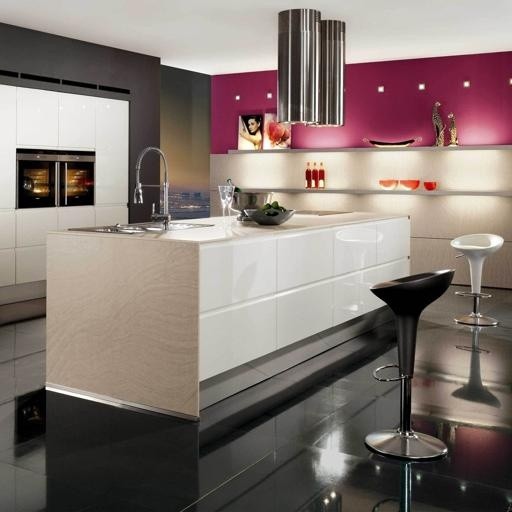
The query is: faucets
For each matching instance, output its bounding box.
[133,146,169,216]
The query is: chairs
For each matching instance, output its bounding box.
[364,269,455,461]
[450,233,504,326]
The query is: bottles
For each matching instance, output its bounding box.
[227,179,243,193]
[303,161,326,190]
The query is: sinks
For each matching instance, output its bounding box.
[68,222,215,234]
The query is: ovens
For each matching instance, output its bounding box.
[16,148,96,210]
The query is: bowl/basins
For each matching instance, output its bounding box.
[400,179,421,192]
[379,179,399,191]
[242,209,298,227]
[227,191,274,222]
[423,182,437,191]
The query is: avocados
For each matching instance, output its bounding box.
[257,201,286,212]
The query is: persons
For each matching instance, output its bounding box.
[240,116,262,150]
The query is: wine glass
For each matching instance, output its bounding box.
[226,186,236,225]
[217,185,229,225]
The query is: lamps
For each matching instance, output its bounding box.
[276,9,345,128]
